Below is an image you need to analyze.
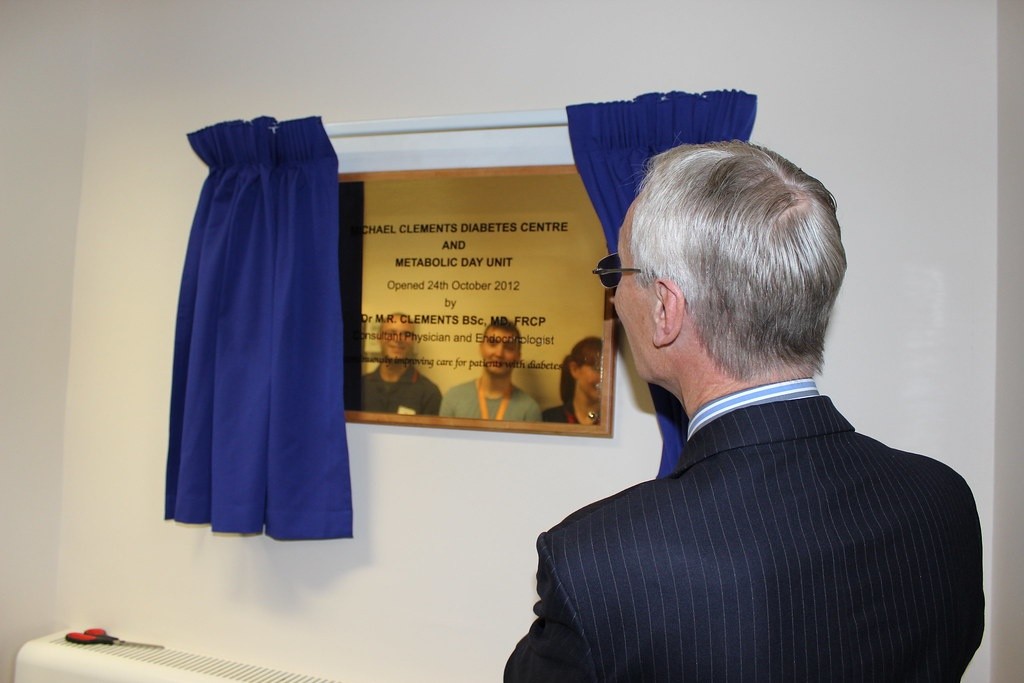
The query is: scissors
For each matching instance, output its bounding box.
[66,627,166,652]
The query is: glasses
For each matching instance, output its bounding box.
[592,252,655,289]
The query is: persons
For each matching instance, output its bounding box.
[438,319,541,422]
[503,143,989,683]
[344,312,443,419]
[545,337,602,428]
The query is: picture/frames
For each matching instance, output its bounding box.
[337,165,614,439]
